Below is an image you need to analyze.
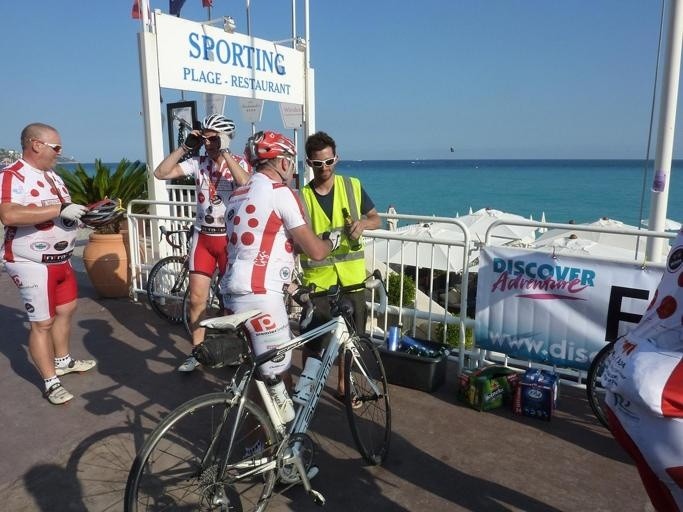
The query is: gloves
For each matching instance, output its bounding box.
[58,203,90,220]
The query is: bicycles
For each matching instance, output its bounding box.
[145,224,230,346]
[123,269,392,511]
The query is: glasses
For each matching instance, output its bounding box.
[30,139,62,153]
[200,135,220,141]
[308,155,337,169]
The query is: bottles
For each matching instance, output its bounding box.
[341,208,362,251]
[405,345,448,356]
[267,375,295,423]
[401,334,429,353]
[387,323,401,352]
[292,351,323,404]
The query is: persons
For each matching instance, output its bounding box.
[153,113,252,374]
[0,122,97,403]
[219,129,342,485]
[597,220,683,512]
[296,130,384,409]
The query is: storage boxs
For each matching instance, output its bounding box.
[515,367,559,422]
[375,335,452,393]
[455,362,519,412]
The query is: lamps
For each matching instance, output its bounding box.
[271,34,307,54]
[198,15,235,35]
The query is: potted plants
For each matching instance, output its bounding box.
[50,158,152,301]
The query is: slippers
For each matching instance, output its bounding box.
[336,390,363,409]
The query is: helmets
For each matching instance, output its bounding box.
[244,131,297,167]
[201,113,237,139]
[79,195,127,230]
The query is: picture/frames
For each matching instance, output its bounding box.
[165,100,199,186]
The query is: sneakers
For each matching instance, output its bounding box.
[262,464,320,485]
[54,358,96,376]
[177,353,200,373]
[43,382,74,405]
